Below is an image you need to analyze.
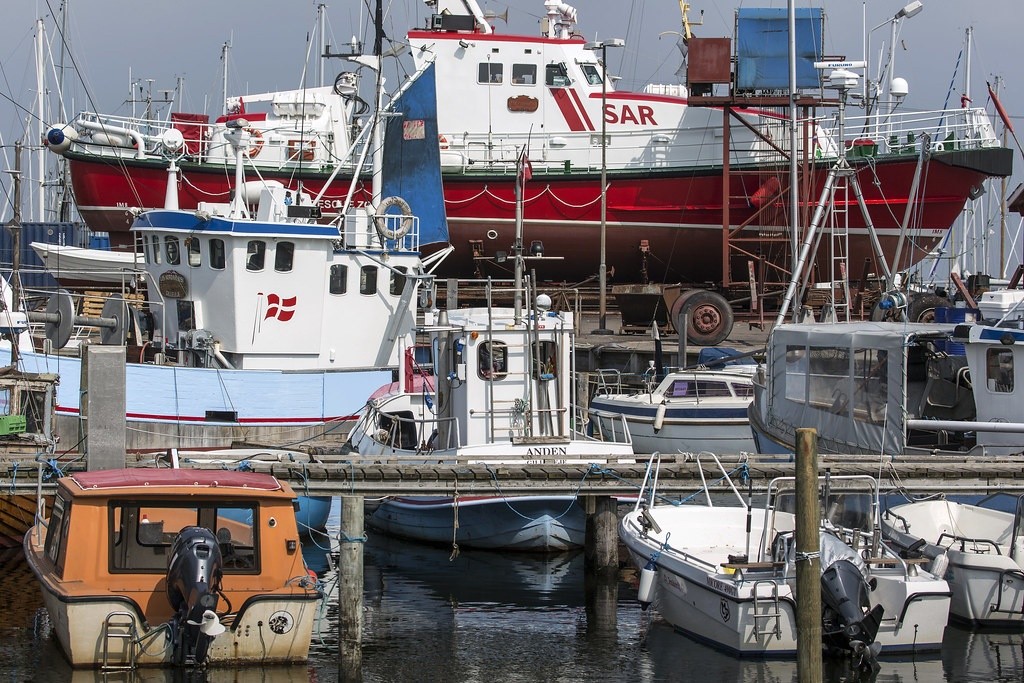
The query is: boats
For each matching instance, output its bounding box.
[22,460,323,672]
[0,0,1024,551]
[873,496,1024,624]
[628,451,951,658]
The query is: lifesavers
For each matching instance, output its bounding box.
[438,134,448,150]
[375,196,412,239]
[235,128,263,157]
